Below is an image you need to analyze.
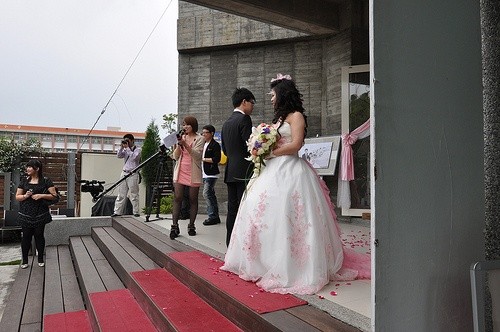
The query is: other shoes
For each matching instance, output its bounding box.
[21,256,29,268]
[37,258,45,267]
[202,218,221,225]
[135,214,139,217]
[170,223,180,239]
[187,224,196,236]
[111,214,121,217]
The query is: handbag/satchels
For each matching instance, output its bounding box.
[38,177,62,205]
[138,172,142,184]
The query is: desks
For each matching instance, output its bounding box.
[91,195,132,216]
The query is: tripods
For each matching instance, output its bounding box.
[146,144,179,222]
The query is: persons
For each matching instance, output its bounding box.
[220,73,342,294]
[15,160,58,269]
[221,87,256,249]
[302,148,319,168]
[111,134,143,217]
[170,116,221,239]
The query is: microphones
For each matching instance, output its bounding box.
[176,134,183,151]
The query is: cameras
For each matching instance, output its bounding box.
[122,140,129,145]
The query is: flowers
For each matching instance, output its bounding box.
[233,123,276,198]
